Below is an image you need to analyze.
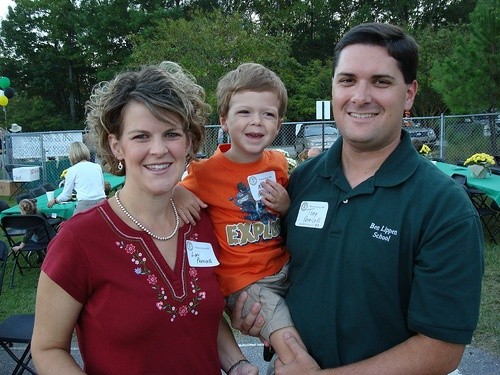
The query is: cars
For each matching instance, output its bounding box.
[403,119,437,146]
[292,123,341,153]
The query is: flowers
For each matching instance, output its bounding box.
[420,144,431,156]
[463,151,496,168]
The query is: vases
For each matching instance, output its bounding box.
[469,165,487,179]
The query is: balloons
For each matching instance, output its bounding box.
[0,76,14,106]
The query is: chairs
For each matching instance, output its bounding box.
[0,177,65,296]
[451,155,500,245]
[0,313,38,375]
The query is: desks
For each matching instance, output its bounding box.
[12,157,70,193]
[434,161,500,241]
[9,179,39,200]
[0,172,126,266]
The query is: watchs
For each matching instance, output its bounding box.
[227,359,250,375]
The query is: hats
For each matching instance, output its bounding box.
[405,111,410,114]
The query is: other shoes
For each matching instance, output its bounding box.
[37,256,46,263]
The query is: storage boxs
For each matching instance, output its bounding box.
[0,179,21,196]
[12,166,40,182]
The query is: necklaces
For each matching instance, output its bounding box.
[115,189,180,241]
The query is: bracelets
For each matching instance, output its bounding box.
[54,198,58,203]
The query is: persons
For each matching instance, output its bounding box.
[223,23,485,375]
[403,111,416,126]
[299,148,322,162]
[172,63,307,366]
[31,61,260,375]
[47,141,108,217]
[104,181,116,199]
[12,198,59,264]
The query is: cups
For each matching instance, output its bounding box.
[46,191,54,202]
